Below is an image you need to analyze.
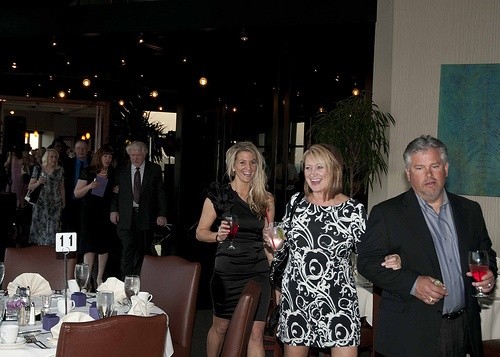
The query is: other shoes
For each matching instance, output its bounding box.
[82,281,90,294]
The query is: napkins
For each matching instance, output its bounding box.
[96,277,127,300]
[129,295,149,316]
[7,273,54,297]
[50,311,96,339]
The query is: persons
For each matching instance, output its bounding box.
[110,141,167,281]
[0,137,92,247]
[74,143,119,287]
[196,142,275,357]
[356,134,498,357]
[271,144,402,357]
[274,151,299,221]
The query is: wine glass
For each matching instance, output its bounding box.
[270,222,285,261]
[225,214,239,249]
[42,170,48,188]
[469,251,490,298]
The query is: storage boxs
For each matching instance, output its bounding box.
[43,315,60,331]
[89,307,99,320]
[71,293,86,307]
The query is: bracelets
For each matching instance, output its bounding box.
[216,234,219,241]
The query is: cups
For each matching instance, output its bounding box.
[68,264,89,306]
[0,262,20,344]
[125,275,153,305]
[41,296,76,331]
[91,290,115,319]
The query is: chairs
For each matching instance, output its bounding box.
[139,255,202,357]
[56,314,167,357]
[0,246,77,292]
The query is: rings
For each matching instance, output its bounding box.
[488,284,491,287]
[429,297,434,302]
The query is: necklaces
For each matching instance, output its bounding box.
[100,171,106,174]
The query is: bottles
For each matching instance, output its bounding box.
[18,301,37,325]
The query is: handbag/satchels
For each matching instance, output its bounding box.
[269,192,305,288]
[24,166,42,204]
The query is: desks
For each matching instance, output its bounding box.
[0,289,174,357]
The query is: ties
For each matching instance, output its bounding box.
[134,168,141,204]
[79,160,84,177]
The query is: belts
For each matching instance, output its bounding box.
[133,207,143,213]
[442,309,465,320]
